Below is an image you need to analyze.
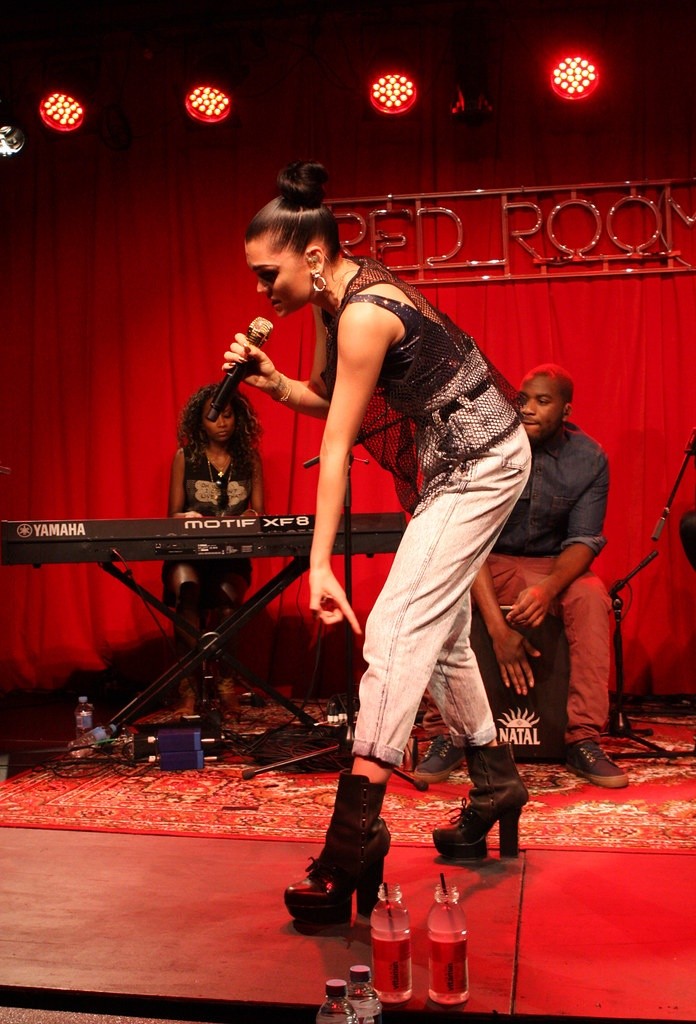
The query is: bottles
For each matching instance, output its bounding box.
[315,882,413,1024]
[67,696,116,758]
[428,884,469,1005]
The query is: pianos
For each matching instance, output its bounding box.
[0,510,407,566]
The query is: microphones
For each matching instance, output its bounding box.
[205,316,273,423]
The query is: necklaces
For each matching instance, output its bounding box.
[207,449,232,486]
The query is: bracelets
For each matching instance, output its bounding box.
[243,509,258,516]
[272,374,292,403]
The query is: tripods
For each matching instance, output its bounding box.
[241,407,430,792]
[597,550,678,760]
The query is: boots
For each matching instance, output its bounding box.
[284,768,390,922]
[432,744,529,860]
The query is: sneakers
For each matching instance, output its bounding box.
[565,745,627,788]
[415,734,465,780]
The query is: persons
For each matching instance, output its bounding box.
[222,160,533,922]
[413,364,630,788]
[164,384,264,720]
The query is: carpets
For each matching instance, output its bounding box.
[0,698,696,854]
